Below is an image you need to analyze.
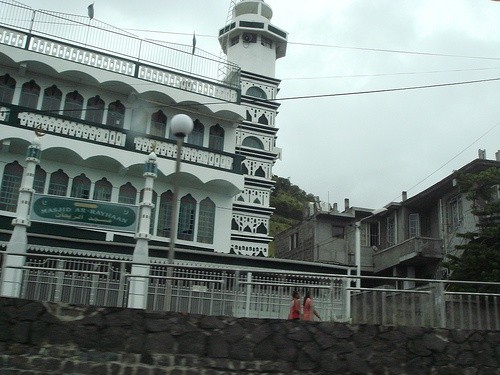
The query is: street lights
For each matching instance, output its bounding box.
[353,208,392,292]
[162,114,194,312]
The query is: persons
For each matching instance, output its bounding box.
[302,290,322,321]
[287,291,303,320]
